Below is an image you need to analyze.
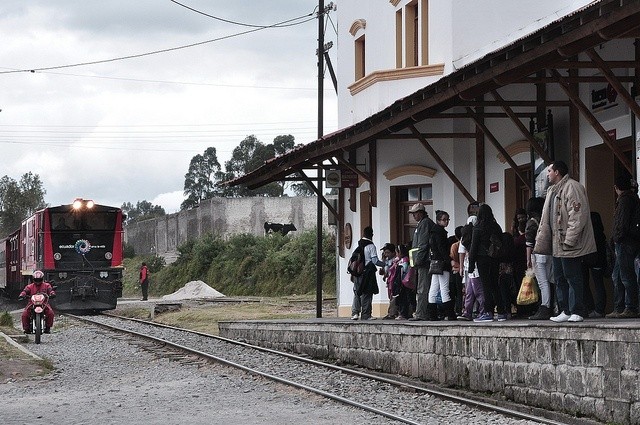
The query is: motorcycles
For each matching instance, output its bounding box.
[18,291,56,343]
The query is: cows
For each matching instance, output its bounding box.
[264,222,297,238]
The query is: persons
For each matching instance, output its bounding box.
[53,216,74,245]
[526,198,553,320]
[534,160,597,321]
[349,224,385,321]
[17,271,57,336]
[137,260,151,301]
[380,201,526,324]
[604,174,640,318]
[583,211,615,318]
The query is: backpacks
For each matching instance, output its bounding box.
[347,240,373,277]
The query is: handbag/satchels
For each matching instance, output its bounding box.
[517,266,538,306]
[427,239,443,274]
[402,268,417,290]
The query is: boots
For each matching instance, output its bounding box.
[425,303,441,321]
[442,299,458,321]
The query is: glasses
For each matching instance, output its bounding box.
[439,219,450,221]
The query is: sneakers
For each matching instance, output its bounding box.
[528,305,556,320]
[473,309,494,322]
[606,308,623,318]
[498,313,507,321]
[361,316,377,320]
[350,314,359,319]
[456,313,471,320]
[567,314,584,322]
[550,311,571,323]
[615,307,640,317]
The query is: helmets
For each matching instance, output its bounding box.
[32,270,44,279]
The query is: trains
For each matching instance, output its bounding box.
[0,197,127,314]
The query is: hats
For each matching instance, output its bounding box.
[408,202,425,213]
[381,243,395,252]
